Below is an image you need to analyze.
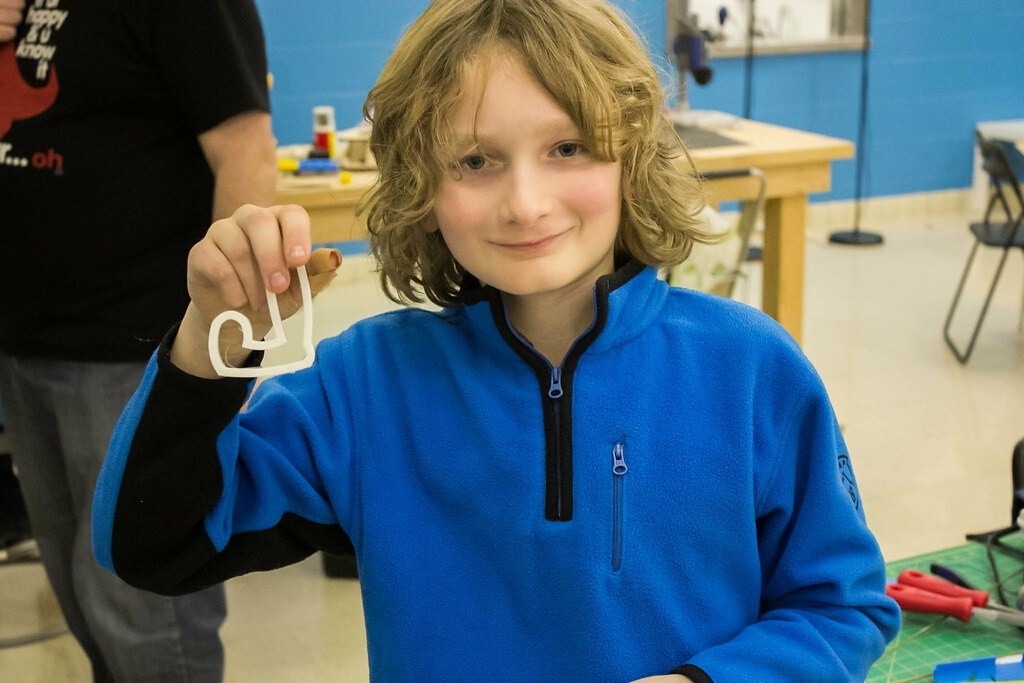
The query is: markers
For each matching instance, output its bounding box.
[277,158,340,172]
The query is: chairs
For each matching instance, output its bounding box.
[944,129,1024,364]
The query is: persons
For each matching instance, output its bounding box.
[90,0,902,682]
[1,0,280,681]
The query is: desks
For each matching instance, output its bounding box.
[968,120,1024,218]
[271,109,855,351]
